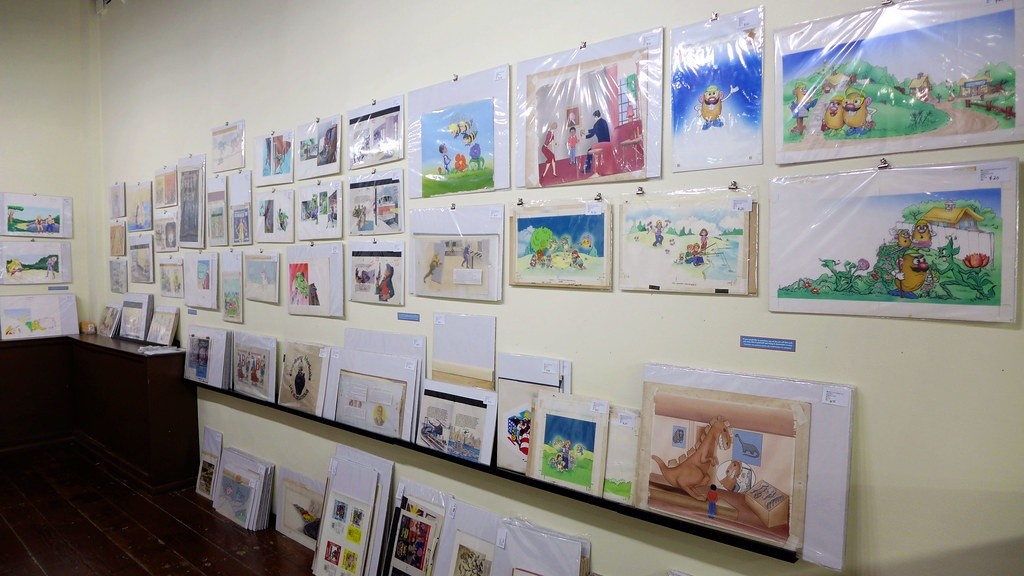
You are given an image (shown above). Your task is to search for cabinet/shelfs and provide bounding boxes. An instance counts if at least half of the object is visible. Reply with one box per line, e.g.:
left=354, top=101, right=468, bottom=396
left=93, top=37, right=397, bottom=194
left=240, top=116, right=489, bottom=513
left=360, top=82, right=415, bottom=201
left=0, top=332, right=200, bottom=494
